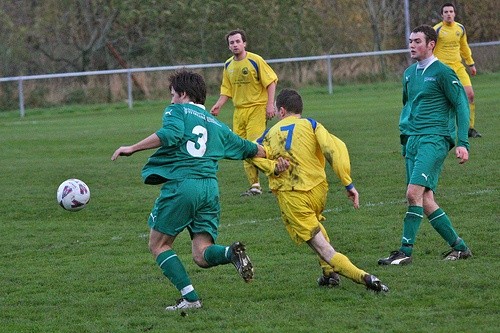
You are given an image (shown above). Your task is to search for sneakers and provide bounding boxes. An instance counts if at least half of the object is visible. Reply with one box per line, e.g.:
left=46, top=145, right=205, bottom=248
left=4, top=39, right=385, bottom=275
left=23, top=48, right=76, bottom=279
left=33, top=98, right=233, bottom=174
left=379, top=251, right=412, bottom=266
left=365, top=274, right=388, bottom=293
left=441, top=248, right=472, bottom=261
left=230, top=241, right=254, bottom=282
left=468, top=129, right=480, bottom=138
left=317, top=270, right=340, bottom=287
left=166, top=299, right=201, bottom=311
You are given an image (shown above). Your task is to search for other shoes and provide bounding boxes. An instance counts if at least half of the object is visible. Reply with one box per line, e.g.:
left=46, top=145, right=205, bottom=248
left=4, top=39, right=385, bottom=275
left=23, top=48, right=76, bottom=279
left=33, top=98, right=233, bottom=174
left=240, top=188, right=262, bottom=198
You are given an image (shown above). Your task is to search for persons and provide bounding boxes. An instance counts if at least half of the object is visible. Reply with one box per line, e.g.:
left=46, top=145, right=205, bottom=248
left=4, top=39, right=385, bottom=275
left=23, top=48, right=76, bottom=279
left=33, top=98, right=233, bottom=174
left=111, top=70, right=266, bottom=312
left=377, top=24, right=474, bottom=266
left=245, top=90, right=389, bottom=294
left=210, top=30, right=278, bottom=197
left=430, top=3, right=482, bottom=138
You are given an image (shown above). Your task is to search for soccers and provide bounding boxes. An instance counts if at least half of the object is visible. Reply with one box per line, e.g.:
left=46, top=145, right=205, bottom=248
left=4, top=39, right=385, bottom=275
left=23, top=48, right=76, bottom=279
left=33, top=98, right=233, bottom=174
left=56, top=178, right=91, bottom=212
